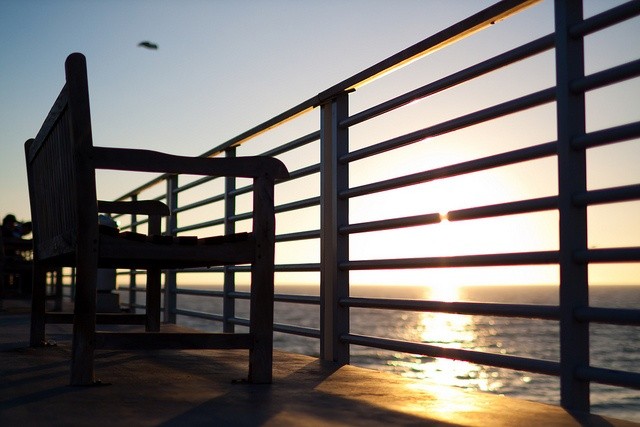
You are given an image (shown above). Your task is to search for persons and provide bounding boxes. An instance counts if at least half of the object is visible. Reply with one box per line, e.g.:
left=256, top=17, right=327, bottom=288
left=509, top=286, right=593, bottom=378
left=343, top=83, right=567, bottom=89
left=0, top=213, right=35, bottom=296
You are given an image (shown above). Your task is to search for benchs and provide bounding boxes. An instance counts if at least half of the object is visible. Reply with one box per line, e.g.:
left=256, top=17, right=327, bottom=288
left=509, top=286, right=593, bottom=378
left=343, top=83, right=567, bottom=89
left=23, top=52, right=289, bottom=391
